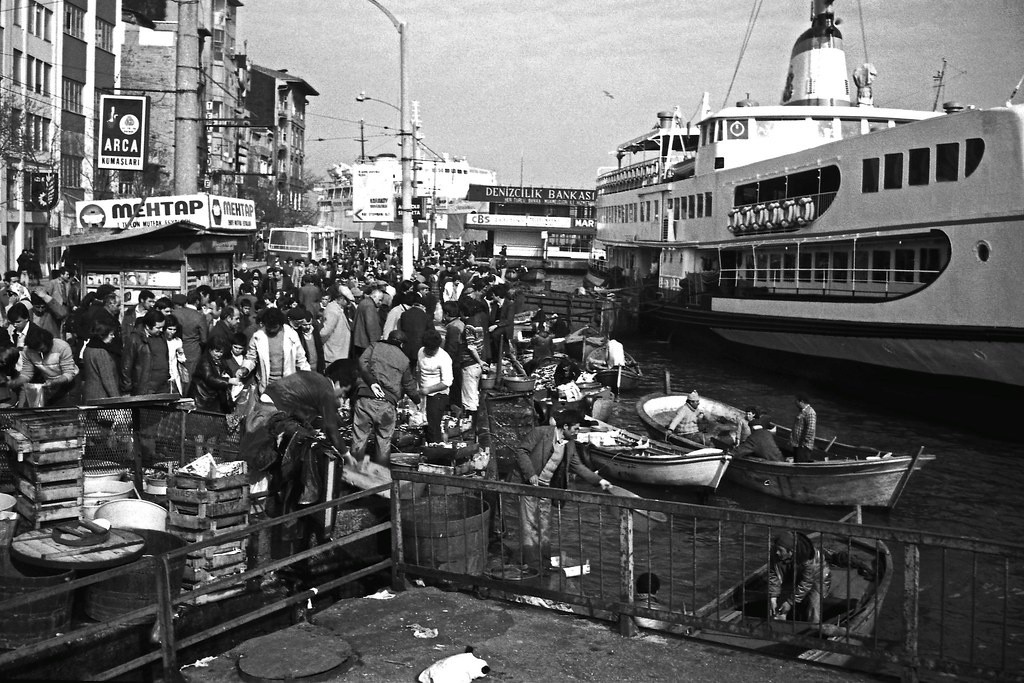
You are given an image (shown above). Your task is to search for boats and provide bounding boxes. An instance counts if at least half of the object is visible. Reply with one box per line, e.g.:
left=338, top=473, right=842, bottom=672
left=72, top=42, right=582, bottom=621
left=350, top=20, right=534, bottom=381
left=580, top=0, right=1023, bottom=444
left=513, top=307, right=734, bottom=495
left=636, top=366, right=937, bottom=515
left=685, top=531, right=894, bottom=667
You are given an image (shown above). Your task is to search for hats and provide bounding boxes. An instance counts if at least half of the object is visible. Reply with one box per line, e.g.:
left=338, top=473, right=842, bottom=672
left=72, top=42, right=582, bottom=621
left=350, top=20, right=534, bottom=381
left=775, top=530, right=794, bottom=550
left=688, top=390, right=699, bottom=401
left=748, top=419, right=762, bottom=426
left=377, top=280, right=389, bottom=286
left=417, top=283, right=430, bottom=289
left=339, top=286, right=355, bottom=302
left=389, top=330, right=408, bottom=342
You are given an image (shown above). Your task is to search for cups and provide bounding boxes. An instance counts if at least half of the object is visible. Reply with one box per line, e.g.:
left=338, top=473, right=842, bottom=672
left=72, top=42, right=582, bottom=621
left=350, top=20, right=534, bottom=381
left=23, top=382, right=49, bottom=408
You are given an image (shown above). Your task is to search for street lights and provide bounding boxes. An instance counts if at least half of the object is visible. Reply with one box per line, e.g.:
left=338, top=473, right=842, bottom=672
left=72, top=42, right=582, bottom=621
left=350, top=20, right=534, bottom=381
left=354, top=0, right=421, bottom=279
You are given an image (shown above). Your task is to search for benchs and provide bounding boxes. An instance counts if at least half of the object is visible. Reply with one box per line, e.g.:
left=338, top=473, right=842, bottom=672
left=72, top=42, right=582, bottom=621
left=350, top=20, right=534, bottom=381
left=746, top=566, right=871, bottom=603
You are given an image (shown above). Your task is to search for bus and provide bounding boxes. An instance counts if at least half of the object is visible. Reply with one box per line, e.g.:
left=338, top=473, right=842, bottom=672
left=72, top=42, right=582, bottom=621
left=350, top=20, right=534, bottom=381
left=267, top=225, right=344, bottom=268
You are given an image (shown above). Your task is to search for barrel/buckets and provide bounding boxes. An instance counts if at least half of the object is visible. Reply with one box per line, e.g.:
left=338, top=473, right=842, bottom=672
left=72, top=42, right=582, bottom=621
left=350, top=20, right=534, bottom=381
left=400, top=492, right=491, bottom=576
left=83, top=524, right=186, bottom=622
left=0, top=510, right=19, bottom=547
left=0, top=545, right=77, bottom=651
left=83, top=479, right=172, bottom=533
left=0, top=492, right=18, bottom=512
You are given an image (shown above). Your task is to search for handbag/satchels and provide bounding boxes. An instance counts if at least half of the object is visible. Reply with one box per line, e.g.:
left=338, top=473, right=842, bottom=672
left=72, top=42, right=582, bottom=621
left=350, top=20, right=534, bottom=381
left=178, top=362, right=189, bottom=383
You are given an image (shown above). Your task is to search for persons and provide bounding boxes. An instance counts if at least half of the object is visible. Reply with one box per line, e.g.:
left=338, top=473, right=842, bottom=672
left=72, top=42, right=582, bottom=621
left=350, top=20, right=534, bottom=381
left=790, top=395, right=817, bottom=462
left=522, top=307, right=581, bottom=385
left=412, top=239, right=508, bottom=284
left=232, top=256, right=342, bottom=318
left=514, top=408, right=613, bottom=570
left=633, top=573, right=670, bottom=611
left=0, top=249, right=126, bottom=462
left=769, top=531, right=833, bottom=624
left=342, top=233, right=402, bottom=283
left=727, top=406, right=785, bottom=462
left=251, top=235, right=269, bottom=262
left=663, top=389, right=710, bottom=444
left=602, top=330, right=625, bottom=369
left=320, top=275, right=515, bottom=378
left=120, top=284, right=311, bottom=460
left=240, top=301, right=482, bottom=548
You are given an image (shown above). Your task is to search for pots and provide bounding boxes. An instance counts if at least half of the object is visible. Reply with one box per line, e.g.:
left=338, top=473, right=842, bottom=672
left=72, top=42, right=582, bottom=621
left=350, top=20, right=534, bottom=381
left=390, top=453, right=428, bottom=471
left=481, top=373, right=496, bottom=389
left=503, top=377, right=535, bottom=391
